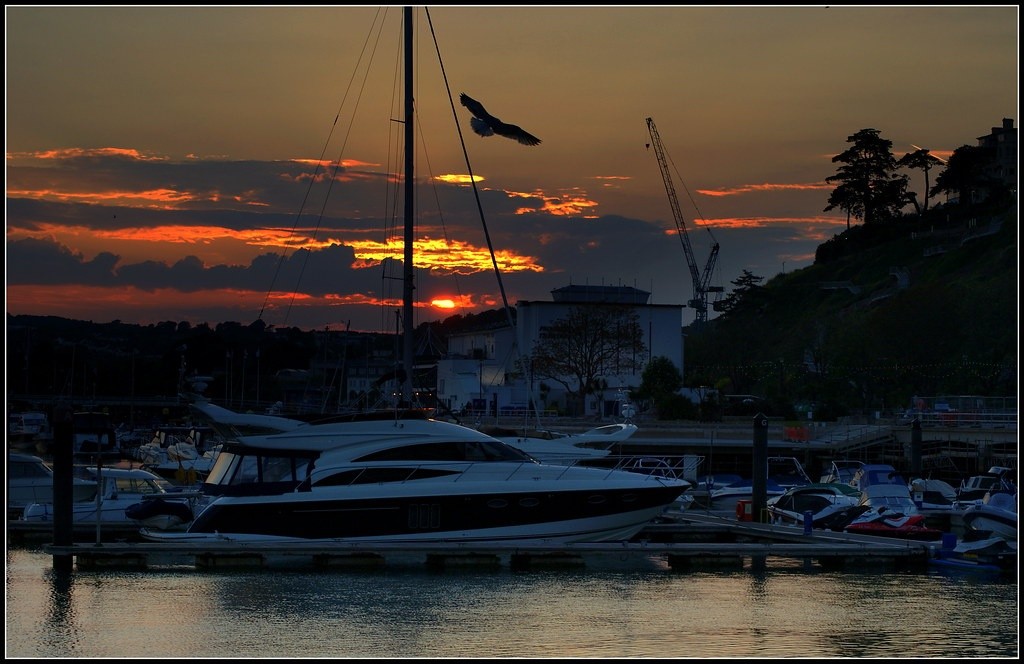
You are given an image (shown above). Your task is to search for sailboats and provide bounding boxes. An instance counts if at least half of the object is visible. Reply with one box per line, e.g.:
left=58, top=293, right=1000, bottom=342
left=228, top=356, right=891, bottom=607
left=4, top=7, right=1018, bottom=578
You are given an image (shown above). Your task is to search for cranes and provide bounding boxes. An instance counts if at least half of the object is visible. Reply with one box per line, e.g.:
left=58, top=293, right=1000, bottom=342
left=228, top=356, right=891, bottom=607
left=646, top=116, right=724, bottom=331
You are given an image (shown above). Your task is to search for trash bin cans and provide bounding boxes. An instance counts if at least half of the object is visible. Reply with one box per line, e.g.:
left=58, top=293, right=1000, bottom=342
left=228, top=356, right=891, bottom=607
left=944, top=412, right=958, bottom=427
left=736, top=501, right=753, bottom=522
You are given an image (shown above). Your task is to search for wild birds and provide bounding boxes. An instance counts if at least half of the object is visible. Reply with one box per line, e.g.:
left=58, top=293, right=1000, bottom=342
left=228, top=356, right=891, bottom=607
left=459, top=91, right=543, bottom=147
